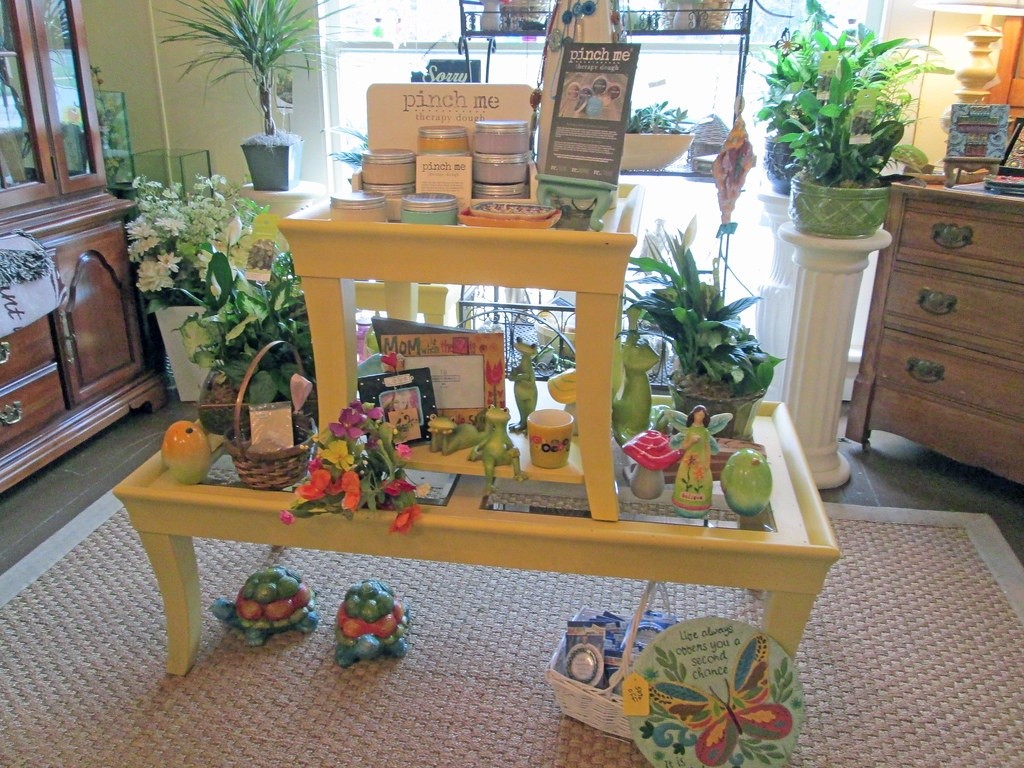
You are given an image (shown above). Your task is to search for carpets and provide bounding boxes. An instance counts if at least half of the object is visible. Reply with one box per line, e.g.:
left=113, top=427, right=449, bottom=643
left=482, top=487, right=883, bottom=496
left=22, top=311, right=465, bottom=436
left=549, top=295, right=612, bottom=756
left=0, top=488, right=1024, bottom=768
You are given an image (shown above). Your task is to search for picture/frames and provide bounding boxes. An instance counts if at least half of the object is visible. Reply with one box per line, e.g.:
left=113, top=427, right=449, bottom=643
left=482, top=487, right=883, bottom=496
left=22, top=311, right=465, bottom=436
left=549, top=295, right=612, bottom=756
left=382, top=334, right=506, bottom=425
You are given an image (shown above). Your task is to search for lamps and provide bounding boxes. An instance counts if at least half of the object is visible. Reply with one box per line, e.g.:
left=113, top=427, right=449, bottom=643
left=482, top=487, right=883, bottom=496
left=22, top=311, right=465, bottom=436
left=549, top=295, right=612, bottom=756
left=913, top=0, right=1024, bottom=184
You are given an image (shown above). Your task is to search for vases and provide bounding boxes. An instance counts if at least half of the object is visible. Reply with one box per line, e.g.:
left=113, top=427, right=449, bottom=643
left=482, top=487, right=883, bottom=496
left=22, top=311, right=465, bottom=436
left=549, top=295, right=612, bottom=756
left=152, top=305, right=211, bottom=403
left=198, top=370, right=318, bottom=438
left=665, top=376, right=766, bottom=444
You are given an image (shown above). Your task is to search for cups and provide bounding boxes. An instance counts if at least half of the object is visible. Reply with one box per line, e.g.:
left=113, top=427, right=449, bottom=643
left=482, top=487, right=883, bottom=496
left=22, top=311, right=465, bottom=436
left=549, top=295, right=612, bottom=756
left=526, top=409, right=574, bottom=468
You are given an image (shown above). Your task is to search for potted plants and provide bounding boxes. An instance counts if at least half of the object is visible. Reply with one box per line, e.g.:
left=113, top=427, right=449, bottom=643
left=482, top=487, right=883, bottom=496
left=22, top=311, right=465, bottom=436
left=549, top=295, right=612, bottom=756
left=619, top=101, right=696, bottom=171
left=152, top=0, right=374, bottom=192
left=752, top=0, right=955, bottom=241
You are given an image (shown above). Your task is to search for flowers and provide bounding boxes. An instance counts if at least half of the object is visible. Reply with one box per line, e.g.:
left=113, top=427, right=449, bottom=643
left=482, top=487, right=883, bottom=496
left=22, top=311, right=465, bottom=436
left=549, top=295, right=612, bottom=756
left=276, top=402, right=429, bottom=535
left=614, top=216, right=787, bottom=397
left=87, top=59, right=127, bottom=185
left=123, top=174, right=314, bottom=402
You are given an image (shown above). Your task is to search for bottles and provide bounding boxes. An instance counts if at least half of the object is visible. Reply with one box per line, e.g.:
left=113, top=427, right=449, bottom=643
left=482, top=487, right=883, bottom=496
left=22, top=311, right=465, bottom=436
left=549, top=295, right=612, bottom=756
left=480, top=0, right=500, bottom=31
left=672, top=0, right=696, bottom=30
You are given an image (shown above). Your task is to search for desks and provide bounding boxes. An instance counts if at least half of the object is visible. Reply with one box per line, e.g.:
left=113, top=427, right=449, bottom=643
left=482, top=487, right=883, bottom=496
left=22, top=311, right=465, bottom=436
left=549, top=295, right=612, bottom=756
left=275, top=178, right=645, bottom=522
left=110, top=400, right=840, bottom=675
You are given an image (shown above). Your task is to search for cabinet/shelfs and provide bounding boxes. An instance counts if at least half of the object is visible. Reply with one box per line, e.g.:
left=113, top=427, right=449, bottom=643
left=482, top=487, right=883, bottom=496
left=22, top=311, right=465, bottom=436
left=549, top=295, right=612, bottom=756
left=457, top=0, right=754, bottom=393
left=843, top=177, right=1024, bottom=486
left=0, top=0, right=167, bottom=499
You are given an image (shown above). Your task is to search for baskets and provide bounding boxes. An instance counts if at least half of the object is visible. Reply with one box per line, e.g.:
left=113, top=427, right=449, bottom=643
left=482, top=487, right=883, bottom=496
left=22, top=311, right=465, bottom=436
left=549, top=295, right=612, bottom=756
left=224, top=341, right=318, bottom=490
left=543, top=579, right=671, bottom=740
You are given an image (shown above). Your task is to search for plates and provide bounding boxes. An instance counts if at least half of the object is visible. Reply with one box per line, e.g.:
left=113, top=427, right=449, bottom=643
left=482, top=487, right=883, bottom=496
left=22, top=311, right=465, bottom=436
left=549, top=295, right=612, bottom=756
left=457, top=202, right=562, bottom=229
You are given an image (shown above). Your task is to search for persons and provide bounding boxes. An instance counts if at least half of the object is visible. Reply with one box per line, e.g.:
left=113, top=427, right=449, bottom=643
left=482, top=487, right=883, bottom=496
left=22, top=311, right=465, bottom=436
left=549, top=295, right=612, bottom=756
left=672, top=404, right=713, bottom=518
